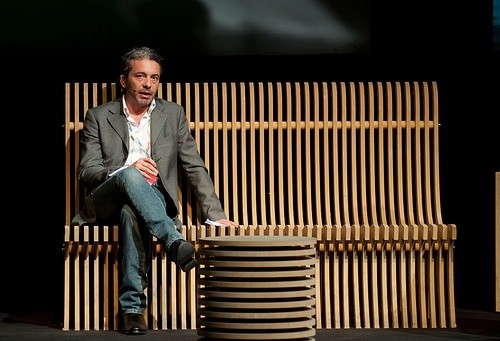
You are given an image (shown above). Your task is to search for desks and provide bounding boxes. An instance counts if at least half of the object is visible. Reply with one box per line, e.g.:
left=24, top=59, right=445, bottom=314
left=196, top=235, right=317, bottom=341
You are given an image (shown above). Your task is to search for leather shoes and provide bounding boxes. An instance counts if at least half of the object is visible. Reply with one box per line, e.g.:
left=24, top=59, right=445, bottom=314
left=121, top=313, right=148, bottom=334
left=171, top=239, right=199, bottom=272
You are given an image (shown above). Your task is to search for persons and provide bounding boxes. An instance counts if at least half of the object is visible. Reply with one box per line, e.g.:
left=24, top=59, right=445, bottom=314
left=79, top=44, right=239, bottom=337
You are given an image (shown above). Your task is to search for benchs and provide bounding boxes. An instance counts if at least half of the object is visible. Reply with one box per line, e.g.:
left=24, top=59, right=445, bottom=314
left=63, top=83, right=458, bottom=333
